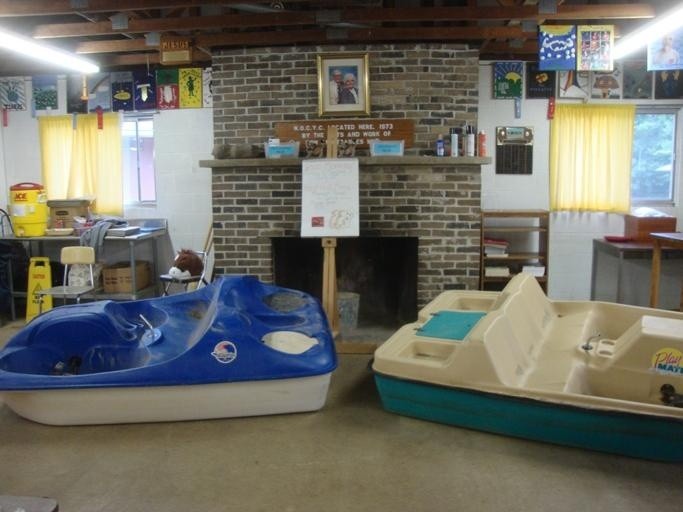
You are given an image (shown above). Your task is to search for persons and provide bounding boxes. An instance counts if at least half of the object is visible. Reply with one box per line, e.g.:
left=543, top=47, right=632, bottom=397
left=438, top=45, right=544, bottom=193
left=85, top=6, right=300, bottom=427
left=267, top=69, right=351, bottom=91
left=330, top=69, right=343, bottom=105
left=671, top=70, right=683, bottom=98
left=653, top=35, right=680, bottom=65
left=186, top=76, right=197, bottom=96
left=339, top=73, right=359, bottom=104
left=656, top=71, right=673, bottom=98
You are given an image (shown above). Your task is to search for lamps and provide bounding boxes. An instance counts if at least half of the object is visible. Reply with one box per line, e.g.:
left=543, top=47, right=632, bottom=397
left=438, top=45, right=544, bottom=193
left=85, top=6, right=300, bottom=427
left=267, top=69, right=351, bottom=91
left=111, top=13, right=129, bottom=30
left=0, top=27, right=100, bottom=75
left=145, top=32, right=162, bottom=46
left=605, top=4, right=683, bottom=62
left=71, top=0, right=88, bottom=9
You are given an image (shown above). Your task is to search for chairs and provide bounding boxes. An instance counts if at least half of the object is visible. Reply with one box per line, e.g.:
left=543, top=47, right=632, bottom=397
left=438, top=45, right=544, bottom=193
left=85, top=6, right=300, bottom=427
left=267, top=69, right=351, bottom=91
left=160, top=249, right=207, bottom=296
left=35, top=246, right=96, bottom=316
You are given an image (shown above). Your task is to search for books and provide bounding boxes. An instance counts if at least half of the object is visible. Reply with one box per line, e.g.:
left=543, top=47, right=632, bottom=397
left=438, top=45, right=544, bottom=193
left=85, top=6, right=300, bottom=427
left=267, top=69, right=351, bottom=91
left=107, top=226, right=140, bottom=236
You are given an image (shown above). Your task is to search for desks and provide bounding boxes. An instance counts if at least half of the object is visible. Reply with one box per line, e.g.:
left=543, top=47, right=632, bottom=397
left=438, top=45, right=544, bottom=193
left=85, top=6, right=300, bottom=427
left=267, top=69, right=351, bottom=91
left=590, top=239, right=683, bottom=311
left=0, top=229, right=165, bottom=320
left=649, top=232, right=683, bottom=312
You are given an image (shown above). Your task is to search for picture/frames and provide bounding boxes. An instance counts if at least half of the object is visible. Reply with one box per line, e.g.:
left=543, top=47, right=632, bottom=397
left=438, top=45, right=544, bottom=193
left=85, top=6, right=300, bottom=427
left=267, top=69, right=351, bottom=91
left=316, top=53, right=371, bottom=117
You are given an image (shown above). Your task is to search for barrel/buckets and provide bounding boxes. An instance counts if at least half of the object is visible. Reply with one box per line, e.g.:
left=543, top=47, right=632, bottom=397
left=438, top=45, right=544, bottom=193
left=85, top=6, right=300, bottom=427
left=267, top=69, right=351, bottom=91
left=10, top=182, right=49, bottom=237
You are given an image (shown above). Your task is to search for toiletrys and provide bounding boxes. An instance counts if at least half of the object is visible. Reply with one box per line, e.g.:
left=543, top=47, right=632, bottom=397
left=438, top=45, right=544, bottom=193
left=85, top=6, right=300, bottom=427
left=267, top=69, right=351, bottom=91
left=435, top=134, right=445, bottom=156
left=450, top=127, right=458, bottom=157
left=478, top=131, right=487, bottom=158
left=464, top=124, right=475, bottom=157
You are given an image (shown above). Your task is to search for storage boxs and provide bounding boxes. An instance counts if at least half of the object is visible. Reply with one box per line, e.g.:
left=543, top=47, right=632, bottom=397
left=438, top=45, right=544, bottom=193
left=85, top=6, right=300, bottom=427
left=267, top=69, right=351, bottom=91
left=101, top=260, right=152, bottom=293
left=50, top=207, right=88, bottom=230
left=367, top=138, right=405, bottom=156
left=263, top=140, right=300, bottom=159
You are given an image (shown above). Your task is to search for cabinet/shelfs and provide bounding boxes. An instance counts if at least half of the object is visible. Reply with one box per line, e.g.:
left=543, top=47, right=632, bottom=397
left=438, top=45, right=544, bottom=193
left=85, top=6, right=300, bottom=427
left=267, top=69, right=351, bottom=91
left=480, top=211, right=549, bottom=297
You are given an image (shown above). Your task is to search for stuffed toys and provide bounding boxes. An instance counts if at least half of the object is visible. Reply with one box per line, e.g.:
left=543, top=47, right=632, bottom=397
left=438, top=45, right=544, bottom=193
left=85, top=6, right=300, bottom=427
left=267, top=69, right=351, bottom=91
left=169, top=248, right=203, bottom=292
